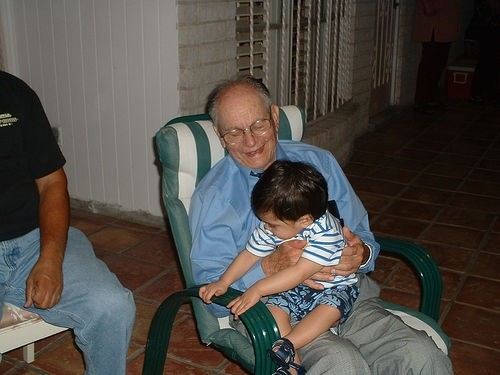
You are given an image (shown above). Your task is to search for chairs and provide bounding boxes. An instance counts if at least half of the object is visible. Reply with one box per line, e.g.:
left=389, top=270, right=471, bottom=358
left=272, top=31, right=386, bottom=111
left=142, top=106, right=451, bottom=375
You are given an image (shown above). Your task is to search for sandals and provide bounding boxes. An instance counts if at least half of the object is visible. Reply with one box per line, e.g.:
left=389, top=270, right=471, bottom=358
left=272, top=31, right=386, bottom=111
left=272, top=362, right=307, bottom=375
left=271, top=338, right=295, bottom=368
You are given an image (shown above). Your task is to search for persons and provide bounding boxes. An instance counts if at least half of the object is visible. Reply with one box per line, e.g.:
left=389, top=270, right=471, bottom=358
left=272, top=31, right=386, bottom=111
left=0, top=70, right=136, bottom=375
left=200, top=160, right=360, bottom=374
left=189, top=74, right=456, bottom=375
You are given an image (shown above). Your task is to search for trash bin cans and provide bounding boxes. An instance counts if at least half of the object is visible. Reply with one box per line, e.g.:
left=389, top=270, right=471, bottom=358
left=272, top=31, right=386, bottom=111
left=446, top=66, right=475, bottom=103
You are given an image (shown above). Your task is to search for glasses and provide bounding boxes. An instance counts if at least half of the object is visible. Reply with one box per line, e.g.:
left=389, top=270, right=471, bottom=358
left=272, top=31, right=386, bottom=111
left=218, top=105, right=272, bottom=145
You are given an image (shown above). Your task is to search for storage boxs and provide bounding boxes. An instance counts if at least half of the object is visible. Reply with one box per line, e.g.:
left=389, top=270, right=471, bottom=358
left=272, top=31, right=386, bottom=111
left=447, top=66, right=475, bottom=99
